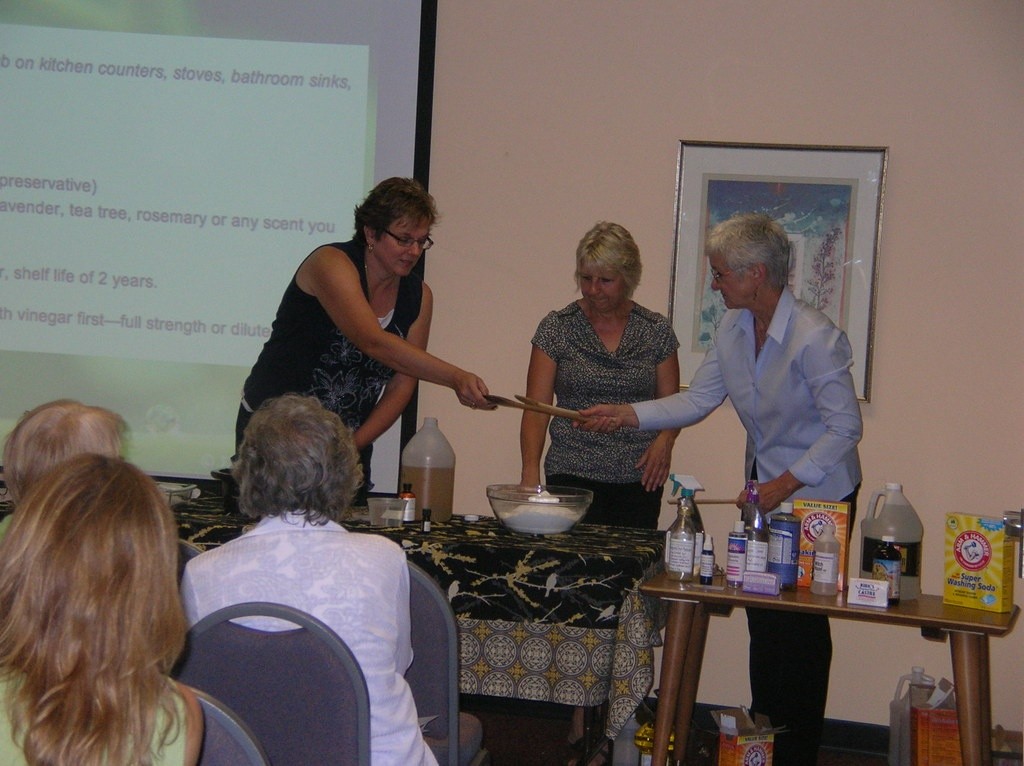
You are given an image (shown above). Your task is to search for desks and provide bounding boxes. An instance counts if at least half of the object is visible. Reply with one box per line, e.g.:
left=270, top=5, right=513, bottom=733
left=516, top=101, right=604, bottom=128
left=0, top=466, right=667, bottom=766
left=641, top=567, right=1019, bottom=766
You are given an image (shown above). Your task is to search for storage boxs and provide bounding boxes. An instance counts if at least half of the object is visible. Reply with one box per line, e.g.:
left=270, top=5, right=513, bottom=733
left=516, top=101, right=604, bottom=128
left=794, top=498, right=851, bottom=590
left=945, top=512, right=1014, bottom=613
left=709, top=704, right=789, bottom=766
left=908, top=677, right=962, bottom=766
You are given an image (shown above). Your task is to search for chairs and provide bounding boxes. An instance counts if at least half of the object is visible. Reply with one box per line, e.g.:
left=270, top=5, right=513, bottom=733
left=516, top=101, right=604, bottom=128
left=170, top=603, right=372, bottom=766
left=404, top=557, right=483, bottom=766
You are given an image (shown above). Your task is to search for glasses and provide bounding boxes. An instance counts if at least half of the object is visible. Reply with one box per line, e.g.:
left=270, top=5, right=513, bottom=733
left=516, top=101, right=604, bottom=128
left=382, top=229, right=433, bottom=250
left=710, top=269, right=745, bottom=281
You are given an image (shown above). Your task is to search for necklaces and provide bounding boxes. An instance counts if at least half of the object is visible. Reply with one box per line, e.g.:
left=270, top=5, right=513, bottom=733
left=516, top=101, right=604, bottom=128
left=364, top=260, right=374, bottom=298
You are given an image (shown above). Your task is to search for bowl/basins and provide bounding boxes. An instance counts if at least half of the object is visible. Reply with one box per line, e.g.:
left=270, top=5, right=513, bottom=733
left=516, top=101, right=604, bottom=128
left=366, top=497, right=409, bottom=527
left=485, top=484, right=594, bottom=534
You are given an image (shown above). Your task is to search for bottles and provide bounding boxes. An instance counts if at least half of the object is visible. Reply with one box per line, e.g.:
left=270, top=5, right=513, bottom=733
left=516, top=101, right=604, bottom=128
left=809, top=524, right=841, bottom=596
left=668, top=490, right=715, bottom=585
left=769, top=502, right=802, bottom=591
left=398, top=482, right=416, bottom=526
left=612, top=698, right=679, bottom=766
left=859, top=481, right=925, bottom=606
left=421, top=508, right=432, bottom=533
left=886, top=664, right=936, bottom=765
left=399, top=416, right=456, bottom=522
left=725, top=520, right=748, bottom=589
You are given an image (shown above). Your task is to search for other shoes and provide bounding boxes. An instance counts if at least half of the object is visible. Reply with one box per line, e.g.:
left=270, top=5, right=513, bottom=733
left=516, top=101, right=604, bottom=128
left=562, top=729, right=602, bottom=766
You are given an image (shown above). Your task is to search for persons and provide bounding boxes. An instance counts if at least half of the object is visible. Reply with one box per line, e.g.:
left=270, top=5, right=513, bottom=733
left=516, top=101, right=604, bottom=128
left=0, top=453, right=204, bottom=766
left=236, top=176, right=497, bottom=493
left=567, top=217, right=863, bottom=766
left=0, top=399, right=132, bottom=540
left=516, top=221, right=680, bottom=766
left=173, top=393, right=439, bottom=766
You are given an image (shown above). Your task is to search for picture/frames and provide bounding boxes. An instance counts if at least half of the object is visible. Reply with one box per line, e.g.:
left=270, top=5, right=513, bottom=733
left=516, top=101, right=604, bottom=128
left=666, top=138, right=891, bottom=404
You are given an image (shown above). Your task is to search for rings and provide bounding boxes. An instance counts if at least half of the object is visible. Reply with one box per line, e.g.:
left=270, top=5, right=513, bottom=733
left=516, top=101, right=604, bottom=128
left=470, top=405, right=477, bottom=408
